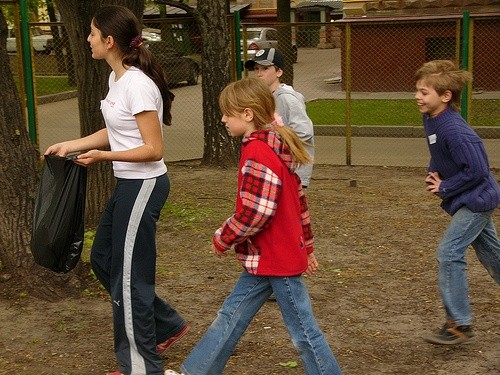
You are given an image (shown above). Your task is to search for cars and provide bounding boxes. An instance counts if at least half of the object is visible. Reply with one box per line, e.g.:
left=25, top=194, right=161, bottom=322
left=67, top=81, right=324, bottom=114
left=6, top=25, right=56, bottom=55
left=141, top=41, right=200, bottom=89
left=140, top=31, right=163, bottom=43
left=239, top=28, right=298, bottom=71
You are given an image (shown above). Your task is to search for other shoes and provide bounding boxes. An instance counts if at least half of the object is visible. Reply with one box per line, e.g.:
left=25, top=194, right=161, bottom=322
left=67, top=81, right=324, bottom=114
left=423, top=322, right=475, bottom=346
left=156, top=323, right=191, bottom=356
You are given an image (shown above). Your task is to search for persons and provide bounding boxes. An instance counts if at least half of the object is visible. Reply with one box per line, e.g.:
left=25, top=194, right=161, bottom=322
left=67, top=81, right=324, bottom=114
left=164, top=77, right=342, bottom=375
left=41, top=4, right=190, bottom=375
left=413, top=59, right=499, bottom=345
left=244, top=48, right=315, bottom=194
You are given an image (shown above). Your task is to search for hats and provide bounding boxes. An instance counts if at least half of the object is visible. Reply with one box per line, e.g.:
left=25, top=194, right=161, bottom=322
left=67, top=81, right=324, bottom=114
left=245, top=48, right=284, bottom=70
